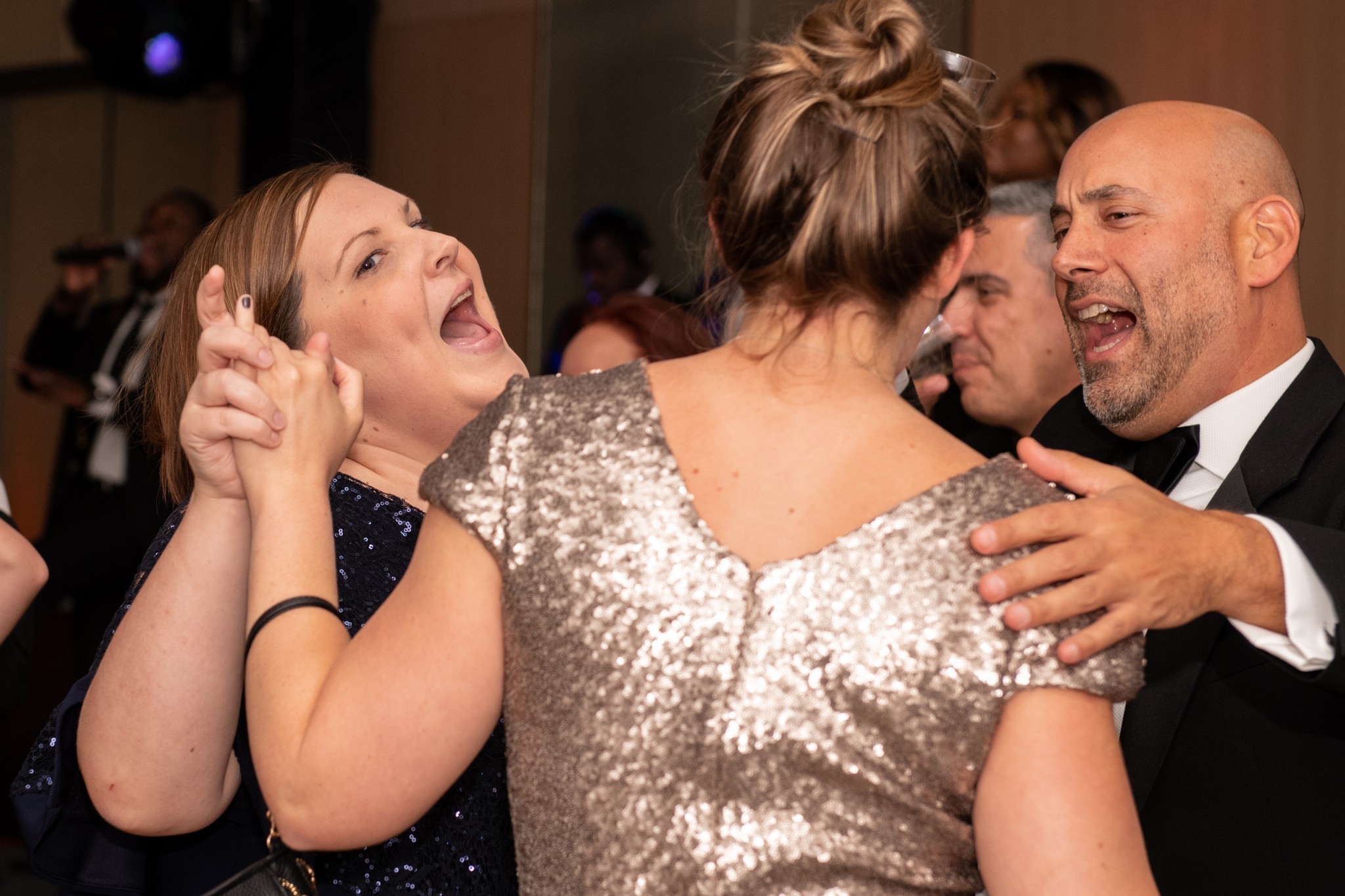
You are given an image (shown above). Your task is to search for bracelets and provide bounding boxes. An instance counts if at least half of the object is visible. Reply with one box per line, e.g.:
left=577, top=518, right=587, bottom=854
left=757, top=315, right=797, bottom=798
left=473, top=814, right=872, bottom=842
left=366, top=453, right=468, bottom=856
left=242, top=596, right=343, bottom=665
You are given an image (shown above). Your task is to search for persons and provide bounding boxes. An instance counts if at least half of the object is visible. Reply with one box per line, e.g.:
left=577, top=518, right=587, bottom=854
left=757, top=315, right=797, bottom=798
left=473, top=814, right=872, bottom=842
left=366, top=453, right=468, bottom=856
left=521, top=61, right=1144, bottom=497
left=1, top=191, right=219, bottom=801
left=232, top=1, right=1161, bottom=895
left=0, top=163, right=532, bottom=896
left=969, top=99, right=1345, bottom=896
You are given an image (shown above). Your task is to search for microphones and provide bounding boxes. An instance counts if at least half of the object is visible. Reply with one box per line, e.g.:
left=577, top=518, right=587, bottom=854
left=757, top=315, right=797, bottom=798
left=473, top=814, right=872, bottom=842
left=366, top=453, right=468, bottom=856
left=53, top=241, right=141, bottom=264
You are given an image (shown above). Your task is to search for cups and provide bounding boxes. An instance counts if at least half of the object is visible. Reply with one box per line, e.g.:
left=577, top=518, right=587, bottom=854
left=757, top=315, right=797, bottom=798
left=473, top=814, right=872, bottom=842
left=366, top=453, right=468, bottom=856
left=905, top=315, right=966, bottom=379
